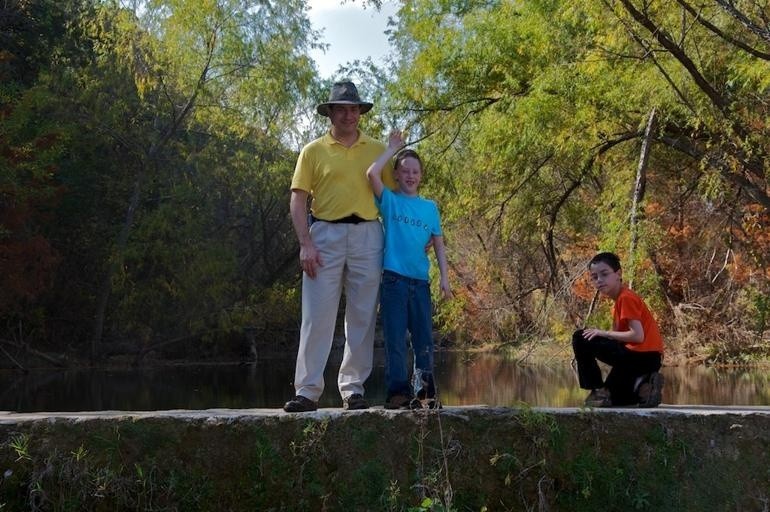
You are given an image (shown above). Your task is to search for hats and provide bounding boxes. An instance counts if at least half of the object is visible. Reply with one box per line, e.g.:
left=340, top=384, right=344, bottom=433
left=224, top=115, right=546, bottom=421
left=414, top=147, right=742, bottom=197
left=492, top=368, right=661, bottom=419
left=315, top=81, right=374, bottom=115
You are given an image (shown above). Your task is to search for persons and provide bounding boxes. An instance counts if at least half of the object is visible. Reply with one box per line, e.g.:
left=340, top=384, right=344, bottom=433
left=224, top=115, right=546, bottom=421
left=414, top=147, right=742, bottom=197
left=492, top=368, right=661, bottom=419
left=366, top=130, right=456, bottom=412
left=570, top=253, right=666, bottom=409
left=282, top=79, right=396, bottom=415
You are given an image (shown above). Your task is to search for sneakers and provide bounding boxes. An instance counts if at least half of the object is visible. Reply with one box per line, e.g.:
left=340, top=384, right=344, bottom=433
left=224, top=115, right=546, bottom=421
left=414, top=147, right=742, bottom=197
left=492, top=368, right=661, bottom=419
left=583, top=388, right=614, bottom=408
left=383, top=392, right=441, bottom=409
left=637, top=371, right=665, bottom=408
left=284, top=395, right=319, bottom=412
left=343, top=394, right=372, bottom=409
